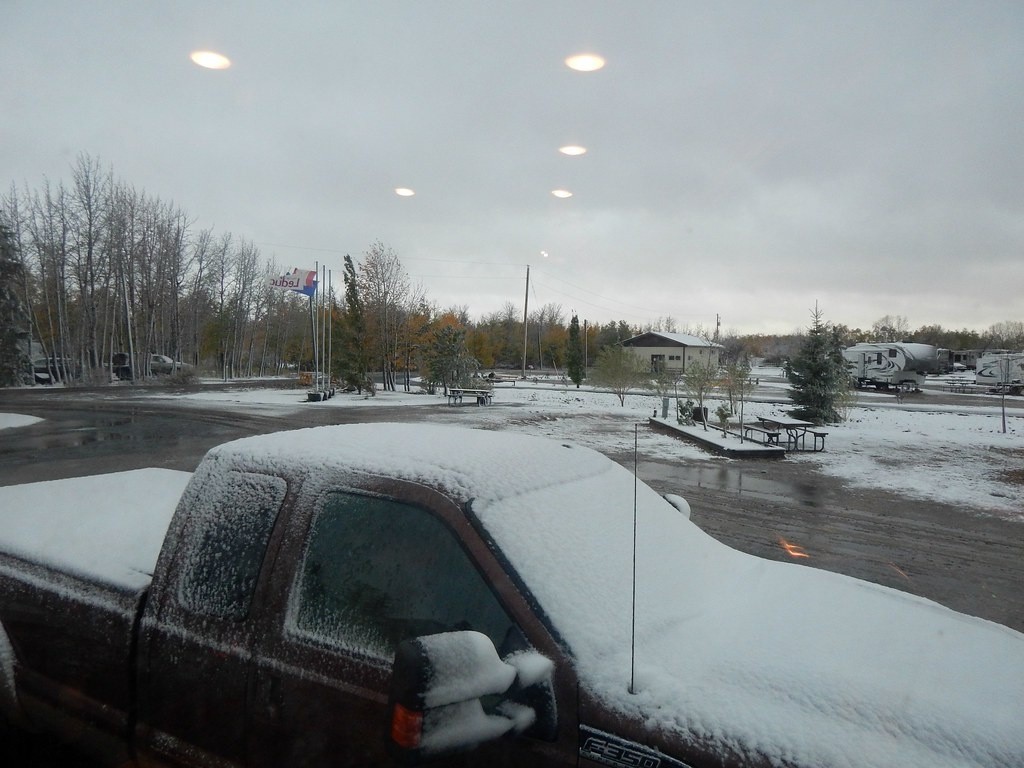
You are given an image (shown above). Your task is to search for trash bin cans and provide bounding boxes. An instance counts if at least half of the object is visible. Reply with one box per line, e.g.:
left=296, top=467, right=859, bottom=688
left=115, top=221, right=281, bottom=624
left=692, top=407, right=708, bottom=421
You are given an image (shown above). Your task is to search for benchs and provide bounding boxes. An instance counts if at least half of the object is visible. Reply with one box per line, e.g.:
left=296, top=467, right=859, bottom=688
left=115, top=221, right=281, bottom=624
left=788, top=427, right=828, bottom=453
left=446, top=394, right=493, bottom=407
left=942, top=386, right=976, bottom=394
left=743, top=424, right=781, bottom=443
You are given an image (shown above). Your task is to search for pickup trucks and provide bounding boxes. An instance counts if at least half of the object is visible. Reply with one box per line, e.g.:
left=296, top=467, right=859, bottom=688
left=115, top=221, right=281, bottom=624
left=0, top=420, right=1024, bottom=768
left=104, top=354, right=195, bottom=382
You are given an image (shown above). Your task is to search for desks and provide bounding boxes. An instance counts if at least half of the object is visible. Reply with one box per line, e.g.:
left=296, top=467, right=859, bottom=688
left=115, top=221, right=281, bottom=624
left=945, top=381, right=974, bottom=393
left=997, top=383, right=1024, bottom=395
left=756, top=416, right=814, bottom=453
left=448, top=389, right=492, bottom=407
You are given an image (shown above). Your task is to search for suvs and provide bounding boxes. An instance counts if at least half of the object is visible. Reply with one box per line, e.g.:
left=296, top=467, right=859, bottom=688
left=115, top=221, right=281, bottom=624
left=23, top=356, right=84, bottom=384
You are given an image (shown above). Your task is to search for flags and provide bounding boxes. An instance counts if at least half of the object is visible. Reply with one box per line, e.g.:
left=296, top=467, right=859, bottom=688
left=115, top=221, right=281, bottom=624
left=265, top=268, right=320, bottom=298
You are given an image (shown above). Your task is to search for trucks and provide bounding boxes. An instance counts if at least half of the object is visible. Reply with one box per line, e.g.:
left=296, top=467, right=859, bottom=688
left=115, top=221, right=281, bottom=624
left=818, top=339, right=1024, bottom=397
